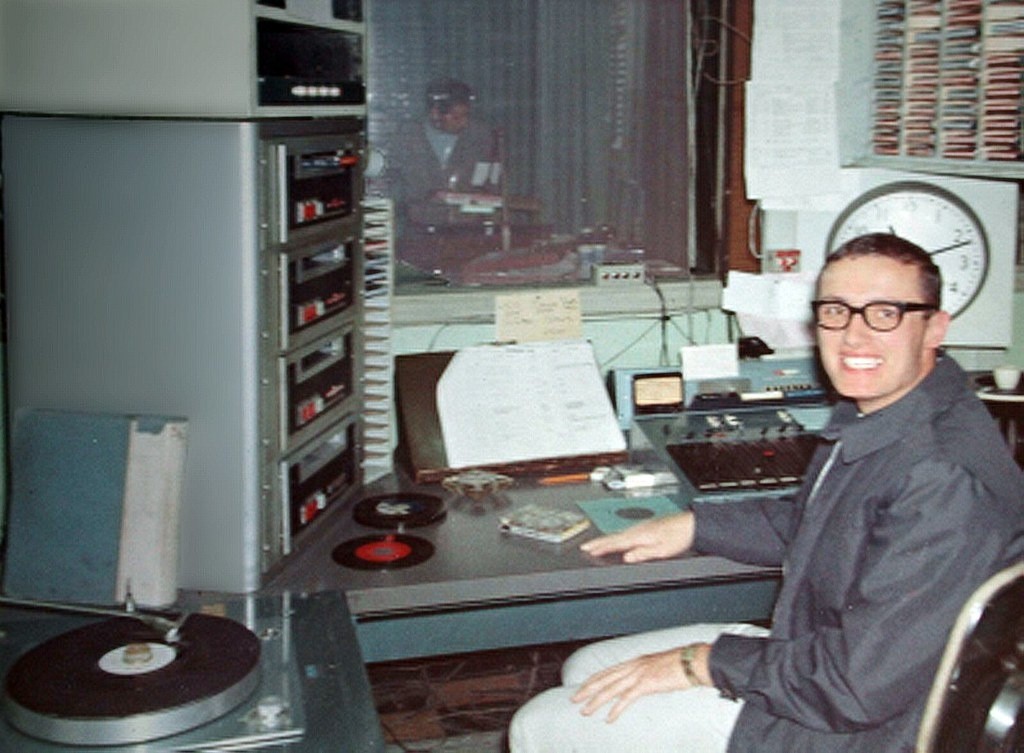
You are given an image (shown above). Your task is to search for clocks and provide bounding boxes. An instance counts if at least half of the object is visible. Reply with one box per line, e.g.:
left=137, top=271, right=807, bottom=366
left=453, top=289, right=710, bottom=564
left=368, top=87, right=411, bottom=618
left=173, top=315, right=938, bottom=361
left=826, top=180, right=991, bottom=321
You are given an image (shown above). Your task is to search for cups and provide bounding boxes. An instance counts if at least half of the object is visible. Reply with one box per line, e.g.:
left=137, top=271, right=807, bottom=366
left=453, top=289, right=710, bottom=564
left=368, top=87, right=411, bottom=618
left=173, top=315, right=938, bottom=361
left=993, top=365, right=1021, bottom=390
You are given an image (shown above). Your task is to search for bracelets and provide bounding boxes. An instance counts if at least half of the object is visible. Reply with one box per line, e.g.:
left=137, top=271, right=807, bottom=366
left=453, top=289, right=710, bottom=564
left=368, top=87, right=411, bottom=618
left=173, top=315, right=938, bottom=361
left=682, top=643, right=705, bottom=686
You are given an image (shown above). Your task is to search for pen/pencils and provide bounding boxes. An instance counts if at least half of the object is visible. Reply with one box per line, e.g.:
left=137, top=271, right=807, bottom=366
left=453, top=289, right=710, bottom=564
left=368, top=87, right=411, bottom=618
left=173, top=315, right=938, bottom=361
left=538, top=475, right=590, bottom=483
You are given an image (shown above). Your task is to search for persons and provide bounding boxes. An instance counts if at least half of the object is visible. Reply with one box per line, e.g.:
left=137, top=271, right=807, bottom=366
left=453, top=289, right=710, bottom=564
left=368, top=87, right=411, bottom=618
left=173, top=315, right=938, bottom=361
left=383, top=79, right=503, bottom=263
left=508, top=232, right=1024, bottom=753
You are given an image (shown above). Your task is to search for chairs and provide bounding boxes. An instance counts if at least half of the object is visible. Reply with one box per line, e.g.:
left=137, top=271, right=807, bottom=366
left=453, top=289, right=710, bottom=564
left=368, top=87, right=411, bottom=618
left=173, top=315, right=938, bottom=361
left=915, top=561, right=1024, bottom=753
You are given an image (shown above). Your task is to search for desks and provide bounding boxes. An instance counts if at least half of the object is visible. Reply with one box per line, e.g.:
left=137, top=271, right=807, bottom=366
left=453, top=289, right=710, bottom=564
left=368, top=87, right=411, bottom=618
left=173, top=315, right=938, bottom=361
left=272, top=473, right=786, bottom=665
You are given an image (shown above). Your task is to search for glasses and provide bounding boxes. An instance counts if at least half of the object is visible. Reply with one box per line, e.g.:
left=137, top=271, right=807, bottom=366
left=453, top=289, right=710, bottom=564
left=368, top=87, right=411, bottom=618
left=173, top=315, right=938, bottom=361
left=810, top=299, right=939, bottom=332
